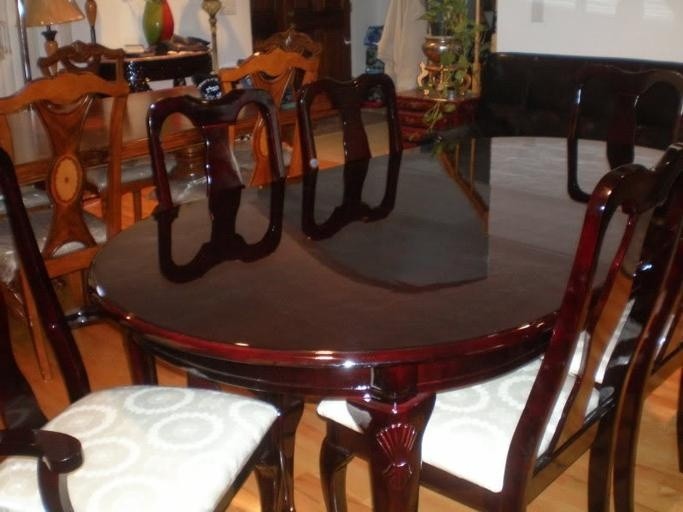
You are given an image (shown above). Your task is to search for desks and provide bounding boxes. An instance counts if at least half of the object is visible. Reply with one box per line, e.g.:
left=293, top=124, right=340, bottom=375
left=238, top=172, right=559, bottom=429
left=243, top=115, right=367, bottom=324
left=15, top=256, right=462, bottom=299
left=0, top=84, right=337, bottom=256
left=98, top=44, right=212, bottom=89
left=88, top=134, right=666, bottom=511
left=397, top=85, right=482, bottom=152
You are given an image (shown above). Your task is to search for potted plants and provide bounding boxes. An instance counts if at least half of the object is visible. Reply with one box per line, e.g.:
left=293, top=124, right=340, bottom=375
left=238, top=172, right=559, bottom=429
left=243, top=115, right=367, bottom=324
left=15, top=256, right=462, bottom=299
left=415, top=3, right=486, bottom=133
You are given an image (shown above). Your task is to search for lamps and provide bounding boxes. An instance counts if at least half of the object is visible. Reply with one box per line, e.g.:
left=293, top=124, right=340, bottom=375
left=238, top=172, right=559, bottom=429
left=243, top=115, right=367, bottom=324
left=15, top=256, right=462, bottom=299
left=24, top=0, right=86, bottom=71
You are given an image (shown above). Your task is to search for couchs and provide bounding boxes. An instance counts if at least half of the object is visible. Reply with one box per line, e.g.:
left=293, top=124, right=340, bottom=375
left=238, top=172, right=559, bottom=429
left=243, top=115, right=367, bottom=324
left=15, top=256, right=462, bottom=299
left=457, top=48, right=683, bottom=153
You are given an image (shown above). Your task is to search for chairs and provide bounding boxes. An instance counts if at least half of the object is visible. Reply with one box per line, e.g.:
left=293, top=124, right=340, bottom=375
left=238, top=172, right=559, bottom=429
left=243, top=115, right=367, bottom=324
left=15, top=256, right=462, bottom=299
left=317, top=148, right=683, bottom=512
left=253, top=31, right=322, bottom=99
left=38, top=41, right=143, bottom=225
left=1, top=71, right=131, bottom=376
left=297, top=71, right=405, bottom=172
left=614, top=200, right=683, bottom=512
left=0, top=147, right=297, bottom=512
left=218, top=50, right=321, bottom=188
left=147, top=87, right=286, bottom=212
